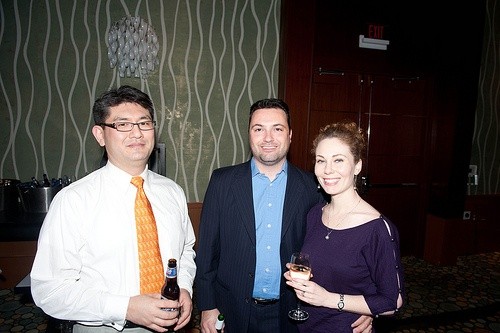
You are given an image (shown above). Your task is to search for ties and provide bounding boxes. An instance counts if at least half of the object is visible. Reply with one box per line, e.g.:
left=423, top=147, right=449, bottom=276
left=130, top=176, right=166, bottom=295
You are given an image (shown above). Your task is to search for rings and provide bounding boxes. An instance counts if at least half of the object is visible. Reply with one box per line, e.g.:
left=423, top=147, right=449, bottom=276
left=302, top=291, right=305, bottom=296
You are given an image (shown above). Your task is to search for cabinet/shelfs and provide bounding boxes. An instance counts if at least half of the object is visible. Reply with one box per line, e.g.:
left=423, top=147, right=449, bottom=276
left=278, top=0, right=479, bottom=256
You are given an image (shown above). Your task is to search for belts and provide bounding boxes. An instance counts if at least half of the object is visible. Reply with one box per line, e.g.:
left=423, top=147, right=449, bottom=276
left=248, top=297, right=279, bottom=305
left=124, top=321, right=140, bottom=328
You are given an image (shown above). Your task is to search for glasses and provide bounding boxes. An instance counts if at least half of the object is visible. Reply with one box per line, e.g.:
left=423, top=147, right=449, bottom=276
left=103, top=121, right=157, bottom=132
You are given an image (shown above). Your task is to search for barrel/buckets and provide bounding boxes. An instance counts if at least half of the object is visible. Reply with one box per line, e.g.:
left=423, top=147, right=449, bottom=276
left=0, top=178, right=21, bottom=214
left=15, top=184, right=63, bottom=213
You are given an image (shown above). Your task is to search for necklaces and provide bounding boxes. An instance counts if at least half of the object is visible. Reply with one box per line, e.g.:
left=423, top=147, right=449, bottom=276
left=326, top=198, right=362, bottom=239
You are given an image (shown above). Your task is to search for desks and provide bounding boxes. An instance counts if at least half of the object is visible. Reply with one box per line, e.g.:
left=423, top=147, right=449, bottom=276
left=0, top=213, right=46, bottom=289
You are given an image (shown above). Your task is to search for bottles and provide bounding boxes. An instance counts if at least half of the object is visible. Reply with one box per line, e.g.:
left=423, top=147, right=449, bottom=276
left=214, top=314, right=225, bottom=333
left=160, top=258, right=180, bottom=333
left=31, top=176, right=78, bottom=187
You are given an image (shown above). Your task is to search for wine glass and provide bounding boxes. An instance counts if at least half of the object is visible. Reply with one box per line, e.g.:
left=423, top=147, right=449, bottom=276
left=288, top=252, right=309, bottom=319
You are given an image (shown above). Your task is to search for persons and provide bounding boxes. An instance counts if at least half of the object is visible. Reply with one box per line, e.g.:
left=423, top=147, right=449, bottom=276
left=284, top=123, right=403, bottom=333
left=193, top=99, right=373, bottom=333
left=30, top=87, right=197, bottom=333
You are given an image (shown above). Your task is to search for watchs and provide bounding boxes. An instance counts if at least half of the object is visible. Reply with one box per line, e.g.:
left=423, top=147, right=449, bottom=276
left=338, top=294, right=344, bottom=310
